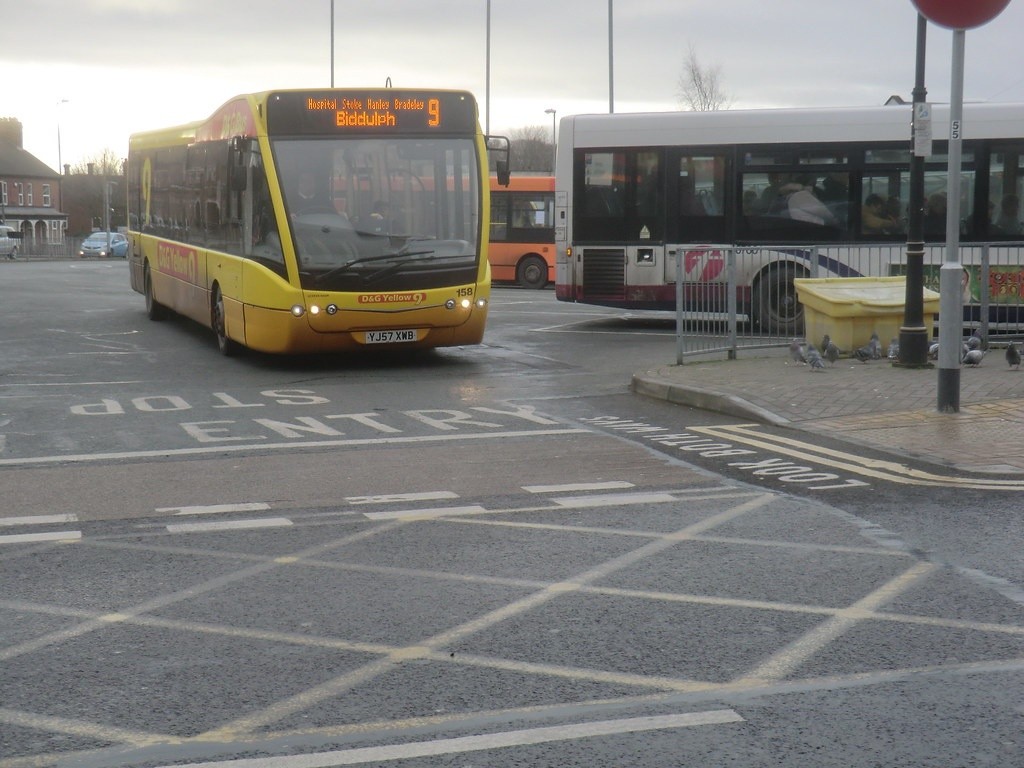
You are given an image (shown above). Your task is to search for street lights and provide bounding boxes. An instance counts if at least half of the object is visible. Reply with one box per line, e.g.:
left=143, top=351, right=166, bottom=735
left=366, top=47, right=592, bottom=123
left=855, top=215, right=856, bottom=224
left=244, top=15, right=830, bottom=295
left=544, top=109, right=556, bottom=178
left=107, top=181, right=118, bottom=258
left=58, top=99, right=69, bottom=212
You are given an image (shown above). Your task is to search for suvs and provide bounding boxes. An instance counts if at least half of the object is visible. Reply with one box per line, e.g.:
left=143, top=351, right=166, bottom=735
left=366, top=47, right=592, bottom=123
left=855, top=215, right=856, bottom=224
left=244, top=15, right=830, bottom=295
left=0, top=226, right=22, bottom=259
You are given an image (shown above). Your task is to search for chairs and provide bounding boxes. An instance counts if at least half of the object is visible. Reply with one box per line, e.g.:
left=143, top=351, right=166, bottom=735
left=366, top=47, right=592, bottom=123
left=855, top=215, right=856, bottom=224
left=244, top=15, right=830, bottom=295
left=586, top=181, right=717, bottom=216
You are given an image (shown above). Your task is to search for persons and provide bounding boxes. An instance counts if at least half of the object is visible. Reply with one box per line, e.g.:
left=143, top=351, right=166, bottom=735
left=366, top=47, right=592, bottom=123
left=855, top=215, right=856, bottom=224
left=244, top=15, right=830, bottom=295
left=861, top=191, right=907, bottom=234
left=753, top=157, right=848, bottom=227
left=289, top=168, right=349, bottom=220
left=924, top=189, right=1023, bottom=236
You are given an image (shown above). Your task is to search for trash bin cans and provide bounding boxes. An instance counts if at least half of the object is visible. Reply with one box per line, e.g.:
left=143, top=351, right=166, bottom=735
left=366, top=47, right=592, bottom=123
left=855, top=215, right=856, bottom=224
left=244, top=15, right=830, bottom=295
left=793, top=276, right=941, bottom=356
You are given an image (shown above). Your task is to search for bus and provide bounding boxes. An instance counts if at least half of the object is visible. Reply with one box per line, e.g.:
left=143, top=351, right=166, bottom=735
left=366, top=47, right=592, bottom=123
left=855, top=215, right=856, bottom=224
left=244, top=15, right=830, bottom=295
left=332, top=171, right=643, bottom=289
left=555, top=104, right=1024, bottom=336
left=127, top=88, right=511, bottom=354
left=91, top=216, right=103, bottom=233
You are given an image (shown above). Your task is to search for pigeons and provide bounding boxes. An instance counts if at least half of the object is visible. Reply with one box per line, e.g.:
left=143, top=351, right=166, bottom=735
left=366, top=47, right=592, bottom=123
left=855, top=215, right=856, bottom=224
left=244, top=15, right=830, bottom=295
left=790, top=331, right=1021, bottom=372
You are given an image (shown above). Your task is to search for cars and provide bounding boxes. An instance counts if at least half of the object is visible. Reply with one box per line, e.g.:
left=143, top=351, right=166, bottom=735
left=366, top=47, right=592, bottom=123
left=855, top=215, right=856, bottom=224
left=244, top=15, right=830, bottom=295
left=79, top=232, right=129, bottom=258
